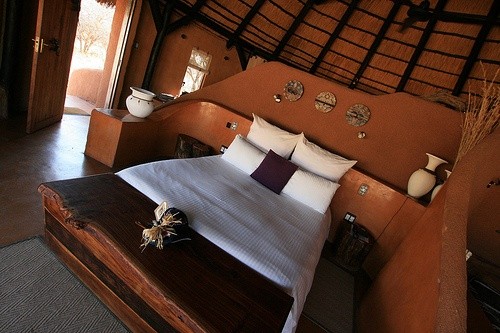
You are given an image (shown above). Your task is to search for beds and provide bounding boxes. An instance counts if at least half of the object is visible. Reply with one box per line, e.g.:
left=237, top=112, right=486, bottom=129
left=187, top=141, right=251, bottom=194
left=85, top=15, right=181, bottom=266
left=115, top=112, right=360, bottom=333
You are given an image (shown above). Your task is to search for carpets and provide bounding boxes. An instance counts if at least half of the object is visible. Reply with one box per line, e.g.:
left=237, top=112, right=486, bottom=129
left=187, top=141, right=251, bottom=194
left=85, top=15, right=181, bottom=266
left=302, top=254, right=355, bottom=333
left=0, top=233, right=130, bottom=333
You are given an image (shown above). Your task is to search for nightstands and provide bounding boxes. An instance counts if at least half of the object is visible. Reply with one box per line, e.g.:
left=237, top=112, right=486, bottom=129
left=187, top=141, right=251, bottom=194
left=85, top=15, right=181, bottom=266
left=84, top=108, right=145, bottom=168
left=321, top=219, right=376, bottom=274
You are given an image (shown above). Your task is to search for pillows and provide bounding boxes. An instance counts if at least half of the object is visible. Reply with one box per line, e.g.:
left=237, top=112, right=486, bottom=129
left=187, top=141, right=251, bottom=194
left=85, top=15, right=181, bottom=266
left=289, top=132, right=358, bottom=183
left=246, top=112, right=301, bottom=160
left=250, top=149, right=299, bottom=195
left=281, top=167, right=341, bottom=215
left=221, top=134, right=267, bottom=175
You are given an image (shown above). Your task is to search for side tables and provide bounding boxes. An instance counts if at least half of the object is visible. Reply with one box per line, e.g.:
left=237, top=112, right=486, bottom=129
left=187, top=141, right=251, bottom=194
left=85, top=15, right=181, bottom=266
left=38, top=172, right=296, bottom=333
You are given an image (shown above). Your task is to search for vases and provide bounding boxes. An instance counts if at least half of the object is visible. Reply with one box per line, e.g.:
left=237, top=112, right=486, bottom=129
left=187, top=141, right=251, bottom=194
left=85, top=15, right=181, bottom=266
left=430, top=169, right=452, bottom=202
left=407, top=152, right=450, bottom=198
left=126, top=86, right=157, bottom=119
